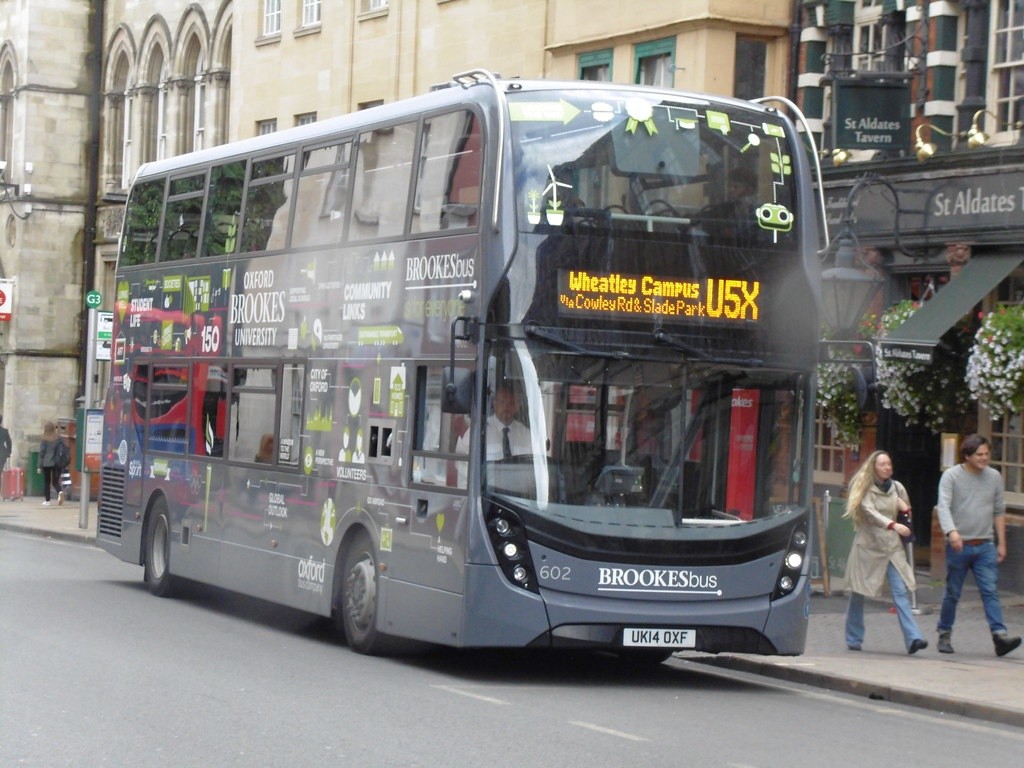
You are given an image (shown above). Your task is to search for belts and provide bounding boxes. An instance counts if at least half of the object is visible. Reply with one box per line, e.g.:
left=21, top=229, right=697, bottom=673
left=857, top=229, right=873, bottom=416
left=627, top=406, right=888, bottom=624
left=963, top=539, right=988, bottom=545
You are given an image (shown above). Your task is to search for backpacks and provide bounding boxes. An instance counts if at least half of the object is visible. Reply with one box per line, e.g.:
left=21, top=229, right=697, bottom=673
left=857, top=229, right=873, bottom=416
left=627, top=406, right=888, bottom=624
left=55, top=438, right=71, bottom=469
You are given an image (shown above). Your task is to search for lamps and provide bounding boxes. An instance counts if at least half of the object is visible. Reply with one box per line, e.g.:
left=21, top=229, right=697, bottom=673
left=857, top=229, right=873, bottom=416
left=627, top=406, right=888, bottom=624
left=832, top=148, right=853, bottom=165
left=914, top=123, right=967, bottom=160
left=967, top=108, right=1023, bottom=149
left=814, top=172, right=925, bottom=346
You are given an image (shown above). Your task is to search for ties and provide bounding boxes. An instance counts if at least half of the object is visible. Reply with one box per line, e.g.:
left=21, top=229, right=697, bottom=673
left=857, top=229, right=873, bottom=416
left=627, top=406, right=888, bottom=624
left=502, top=427, right=512, bottom=457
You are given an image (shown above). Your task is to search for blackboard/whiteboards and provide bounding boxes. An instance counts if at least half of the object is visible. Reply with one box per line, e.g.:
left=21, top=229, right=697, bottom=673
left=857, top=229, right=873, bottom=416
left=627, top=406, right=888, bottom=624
left=767, top=498, right=831, bottom=584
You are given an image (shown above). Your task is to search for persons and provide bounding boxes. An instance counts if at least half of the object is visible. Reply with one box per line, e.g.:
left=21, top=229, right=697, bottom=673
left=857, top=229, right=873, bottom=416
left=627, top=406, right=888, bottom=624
left=844, top=449, right=928, bottom=654
left=454, top=381, right=533, bottom=490
left=0, top=415, right=12, bottom=475
left=241, top=434, right=292, bottom=521
left=936, top=434, right=1022, bottom=656
left=36, top=422, right=64, bottom=506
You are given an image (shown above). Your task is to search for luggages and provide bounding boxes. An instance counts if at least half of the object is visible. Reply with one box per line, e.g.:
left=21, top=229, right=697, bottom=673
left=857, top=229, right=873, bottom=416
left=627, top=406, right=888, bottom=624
left=1, top=454, right=24, bottom=502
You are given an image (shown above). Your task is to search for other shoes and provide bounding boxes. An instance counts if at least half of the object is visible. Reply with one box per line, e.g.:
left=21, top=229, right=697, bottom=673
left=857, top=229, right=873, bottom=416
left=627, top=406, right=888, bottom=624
left=908, top=639, right=928, bottom=654
left=58, top=491, right=64, bottom=505
left=992, top=634, right=1022, bottom=657
left=41, top=501, right=50, bottom=506
left=936, top=633, right=954, bottom=653
left=848, top=645, right=862, bottom=651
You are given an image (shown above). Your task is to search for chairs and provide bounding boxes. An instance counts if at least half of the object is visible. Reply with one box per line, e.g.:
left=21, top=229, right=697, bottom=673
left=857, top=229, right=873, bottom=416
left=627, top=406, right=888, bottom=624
left=261, top=434, right=273, bottom=460
left=150, top=199, right=761, bottom=266
left=604, top=450, right=651, bottom=491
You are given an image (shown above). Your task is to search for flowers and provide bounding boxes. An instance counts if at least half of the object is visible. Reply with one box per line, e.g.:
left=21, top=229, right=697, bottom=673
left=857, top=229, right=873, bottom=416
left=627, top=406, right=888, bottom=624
left=799, top=300, right=1024, bottom=448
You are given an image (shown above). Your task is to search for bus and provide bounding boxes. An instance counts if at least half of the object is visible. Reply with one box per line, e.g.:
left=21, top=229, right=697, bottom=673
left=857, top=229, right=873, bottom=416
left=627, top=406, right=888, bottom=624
left=95, top=68, right=890, bottom=664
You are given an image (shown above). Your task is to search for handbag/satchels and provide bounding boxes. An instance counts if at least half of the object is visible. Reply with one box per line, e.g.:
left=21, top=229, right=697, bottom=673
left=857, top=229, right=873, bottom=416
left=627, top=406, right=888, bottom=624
left=894, top=480, right=916, bottom=544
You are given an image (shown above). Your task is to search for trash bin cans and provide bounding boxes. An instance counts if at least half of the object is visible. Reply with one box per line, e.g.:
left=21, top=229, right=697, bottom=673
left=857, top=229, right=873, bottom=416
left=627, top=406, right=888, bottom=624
left=27, top=449, right=46, bottom=497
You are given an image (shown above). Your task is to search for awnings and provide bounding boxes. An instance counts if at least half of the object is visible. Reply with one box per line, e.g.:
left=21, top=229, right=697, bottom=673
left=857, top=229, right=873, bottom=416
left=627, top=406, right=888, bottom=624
left=880, top=250, right=1024, bottom=365
